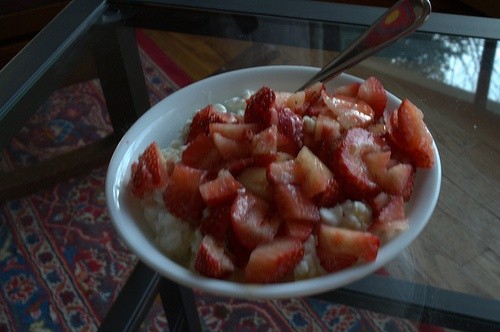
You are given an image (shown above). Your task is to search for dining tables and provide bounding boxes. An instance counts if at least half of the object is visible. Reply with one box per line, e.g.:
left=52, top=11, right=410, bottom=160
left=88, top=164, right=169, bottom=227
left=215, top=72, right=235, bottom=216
left=0, top=1, right=500, bottom=332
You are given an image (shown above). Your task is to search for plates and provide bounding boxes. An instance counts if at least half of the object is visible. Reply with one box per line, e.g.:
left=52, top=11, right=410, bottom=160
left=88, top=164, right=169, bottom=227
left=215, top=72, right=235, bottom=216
left=103, top=64, right=442, bottom=298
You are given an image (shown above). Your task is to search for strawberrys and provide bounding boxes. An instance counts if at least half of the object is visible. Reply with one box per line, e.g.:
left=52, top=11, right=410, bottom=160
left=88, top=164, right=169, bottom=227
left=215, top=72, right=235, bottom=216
left=129, top=77, right=435, bottom=284
